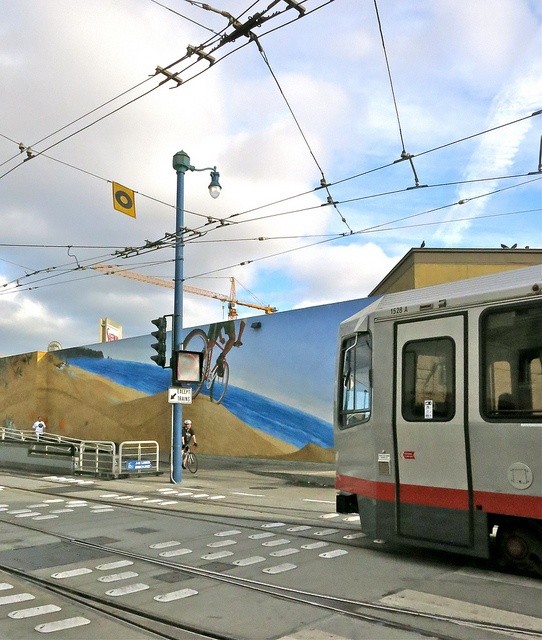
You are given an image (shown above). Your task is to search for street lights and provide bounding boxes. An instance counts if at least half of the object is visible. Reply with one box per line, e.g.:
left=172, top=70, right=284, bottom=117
left=168, top=148, right=224, bottom=483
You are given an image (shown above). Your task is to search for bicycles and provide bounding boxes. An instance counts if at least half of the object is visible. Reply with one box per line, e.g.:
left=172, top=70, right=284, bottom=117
left=168, top=442, right=198, bottom=473
left=180, top=329, right=242, bottom=405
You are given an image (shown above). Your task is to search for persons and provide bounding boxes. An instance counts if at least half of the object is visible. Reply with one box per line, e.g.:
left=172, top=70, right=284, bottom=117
left=181, top=419, right=197, bottom=470
left=32, top=416, right=47, bottom=442
left=201, top=319, right=247, bottom=377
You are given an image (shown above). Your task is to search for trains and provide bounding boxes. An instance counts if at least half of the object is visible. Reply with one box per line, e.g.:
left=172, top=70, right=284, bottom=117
left=326, top=262, right=542, bottom=579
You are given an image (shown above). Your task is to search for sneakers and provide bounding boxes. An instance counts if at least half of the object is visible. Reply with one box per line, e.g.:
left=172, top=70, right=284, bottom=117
left=216, top=353, right=225, bottom=377
left=182, top=462, right=187, bottom=470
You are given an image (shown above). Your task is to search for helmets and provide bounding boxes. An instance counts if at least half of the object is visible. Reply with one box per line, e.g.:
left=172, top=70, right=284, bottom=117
left=183, top=419, right=192, bottom=425
